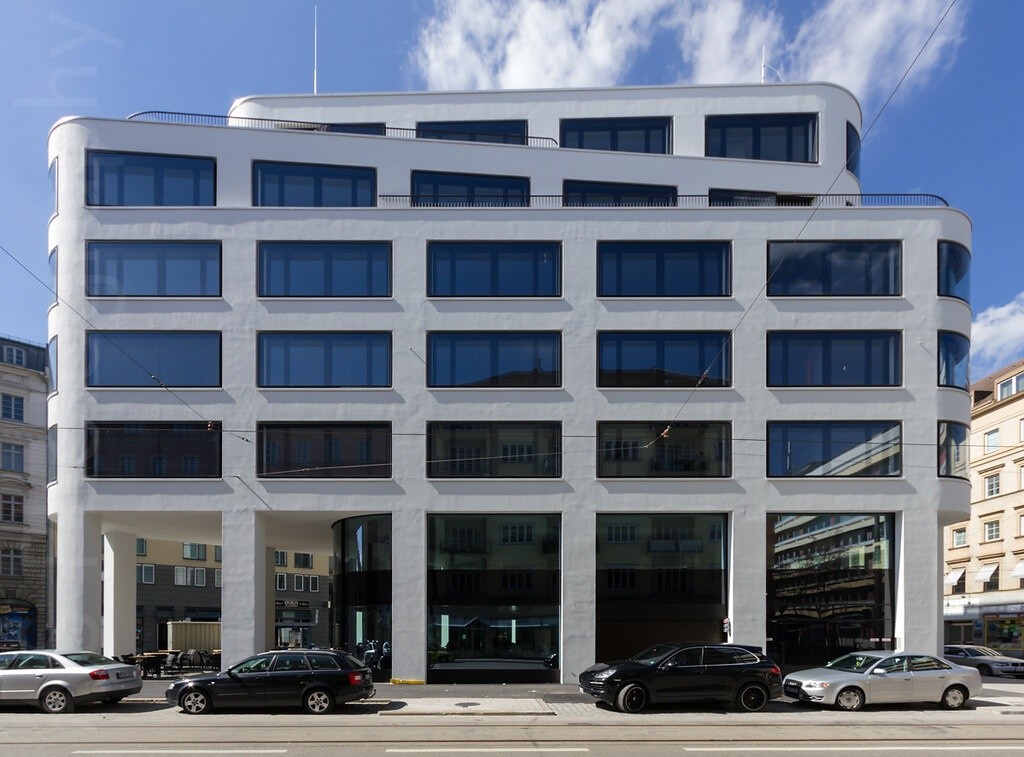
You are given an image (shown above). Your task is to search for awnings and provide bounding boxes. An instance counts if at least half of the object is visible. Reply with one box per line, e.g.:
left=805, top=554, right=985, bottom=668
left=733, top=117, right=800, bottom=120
left=944, top=568, right=966, bottom=586
left=974, top=563, right=998, bottom=582
left=1011, top=559, right=1024, bottom=578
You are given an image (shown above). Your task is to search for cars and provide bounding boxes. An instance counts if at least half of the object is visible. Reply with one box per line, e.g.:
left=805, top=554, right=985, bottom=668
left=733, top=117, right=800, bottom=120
left=543, top=647, right=559, bottom=669
left=943, top=645, right=1024, bottom=679
left=0, top=648, right=143, bottom=714
left=578, top=640, right=787, bottom=714
left=781, top=649, right=984, bottom=712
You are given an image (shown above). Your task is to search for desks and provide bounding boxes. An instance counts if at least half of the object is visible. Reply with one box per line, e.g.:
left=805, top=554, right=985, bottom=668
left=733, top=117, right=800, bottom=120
left=144, top=653, right=169, bottom=680
left=159, top=650, right=181, bottom=657
left=213, top=650, right=222, bottom=654
left=131, top=656, right=156, bottom=680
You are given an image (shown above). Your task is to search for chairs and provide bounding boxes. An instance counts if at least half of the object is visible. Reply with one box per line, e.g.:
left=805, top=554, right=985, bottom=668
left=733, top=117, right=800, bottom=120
left=112, top=648, right=221, bottom=680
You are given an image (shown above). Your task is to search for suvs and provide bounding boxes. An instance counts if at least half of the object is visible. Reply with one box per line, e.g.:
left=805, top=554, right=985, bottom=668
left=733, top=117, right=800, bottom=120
left=164, top=648, right=377, bottom=713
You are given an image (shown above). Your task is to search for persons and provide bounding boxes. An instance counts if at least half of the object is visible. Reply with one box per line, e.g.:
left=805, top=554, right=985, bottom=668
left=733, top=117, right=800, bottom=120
left=492, top=634, right=549, bottom=657
